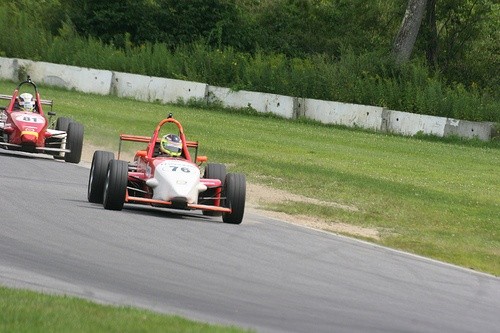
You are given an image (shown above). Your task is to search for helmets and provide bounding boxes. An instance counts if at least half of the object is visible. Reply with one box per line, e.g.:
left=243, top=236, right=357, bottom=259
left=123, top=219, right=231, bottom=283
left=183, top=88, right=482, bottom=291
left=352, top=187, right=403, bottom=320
left=158, top=134, right=182, bottom=157
left=17, top=93, right=36, bottom=112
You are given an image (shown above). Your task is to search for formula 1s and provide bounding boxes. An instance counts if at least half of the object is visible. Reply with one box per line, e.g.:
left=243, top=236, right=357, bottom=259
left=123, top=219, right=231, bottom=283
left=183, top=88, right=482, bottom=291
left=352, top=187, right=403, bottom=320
left=87, top=113, right=247, bottom=224
left=0, top=74, right=85, bottom=164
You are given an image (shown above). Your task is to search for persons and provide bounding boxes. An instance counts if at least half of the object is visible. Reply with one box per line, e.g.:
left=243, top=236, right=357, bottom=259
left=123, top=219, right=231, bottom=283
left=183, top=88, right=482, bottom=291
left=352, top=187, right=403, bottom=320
left=157, top=134, right=183, bottom=157
left=17, top=93, right=37, bottom=112
